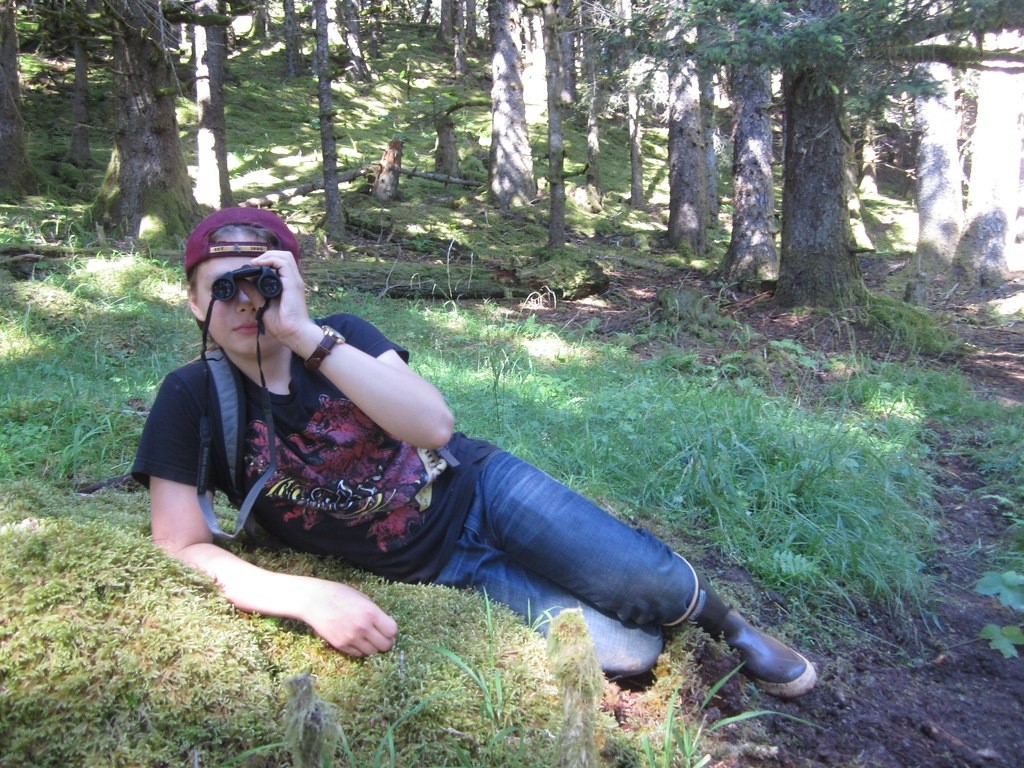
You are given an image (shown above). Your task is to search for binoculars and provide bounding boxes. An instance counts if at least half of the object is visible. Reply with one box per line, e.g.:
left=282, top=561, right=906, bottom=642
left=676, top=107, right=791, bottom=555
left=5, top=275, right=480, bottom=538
left=210, top=264, right=284, bottom=302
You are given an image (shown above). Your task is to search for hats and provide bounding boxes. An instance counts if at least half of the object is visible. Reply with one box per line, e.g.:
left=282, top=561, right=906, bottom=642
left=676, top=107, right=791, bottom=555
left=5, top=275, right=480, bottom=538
left=185, top=207, right=301, bottom=273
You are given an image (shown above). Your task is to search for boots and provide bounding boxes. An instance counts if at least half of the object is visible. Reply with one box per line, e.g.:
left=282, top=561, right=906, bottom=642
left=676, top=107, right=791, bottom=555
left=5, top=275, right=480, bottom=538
left=662, top=552, right=818, bottom=699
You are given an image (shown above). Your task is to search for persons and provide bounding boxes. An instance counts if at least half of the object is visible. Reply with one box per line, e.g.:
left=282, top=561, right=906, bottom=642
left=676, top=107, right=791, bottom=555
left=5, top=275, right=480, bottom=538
left=130, top=207, right=817, bottom=697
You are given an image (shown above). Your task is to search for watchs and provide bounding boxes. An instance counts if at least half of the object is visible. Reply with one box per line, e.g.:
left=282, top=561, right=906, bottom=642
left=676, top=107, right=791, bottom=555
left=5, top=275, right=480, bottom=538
left=303, top=325, right=345, bottom=372
